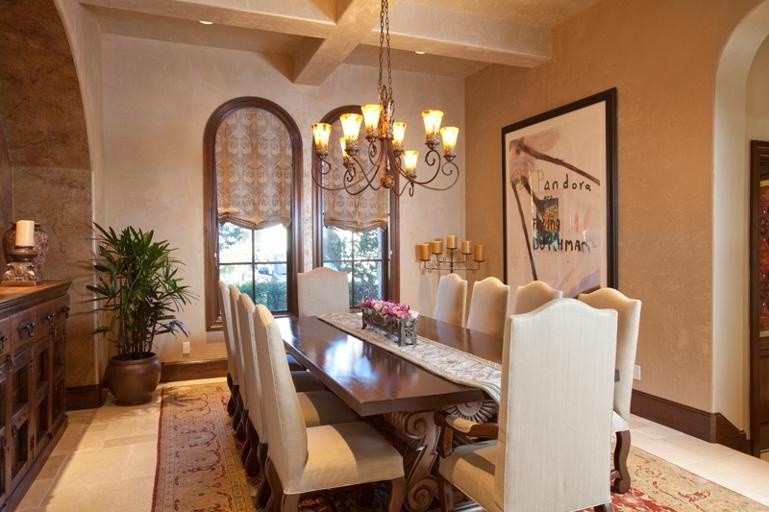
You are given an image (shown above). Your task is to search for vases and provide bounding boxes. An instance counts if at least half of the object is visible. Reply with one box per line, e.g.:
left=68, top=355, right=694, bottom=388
left=4, top=221, right=50, bottom=282
left=362, top=310, right=416, bottom=345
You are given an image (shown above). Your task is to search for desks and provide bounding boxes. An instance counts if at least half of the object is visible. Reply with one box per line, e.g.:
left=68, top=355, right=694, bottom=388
left=279, top=306, right=540, bottom=512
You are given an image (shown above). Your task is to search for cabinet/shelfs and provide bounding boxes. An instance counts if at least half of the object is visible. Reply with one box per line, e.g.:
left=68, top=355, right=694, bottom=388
left=0, top=278, right=73, bottom=510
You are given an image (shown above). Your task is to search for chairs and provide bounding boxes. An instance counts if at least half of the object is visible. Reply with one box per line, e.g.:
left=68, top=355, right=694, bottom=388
left=579, top=283, right=646, bottom=494
left=512, top=281, right=563, bottom=333
left=216, top=276, right=307, bottom=414
left=251, top=304, right=402, bottom=510
left=226, top=285, right=324, bottom=436
left=467, top=276, right=511, bottom=336
left=435, top=296, right=621, bottom=510
left=433, top=272, right=468, bottom=328
left=239, top=296, right=355, bottom=470
left=295, top=267, right=350, bottom=306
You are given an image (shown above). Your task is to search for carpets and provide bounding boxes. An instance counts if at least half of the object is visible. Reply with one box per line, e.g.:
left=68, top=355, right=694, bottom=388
left=150, top=377, right=769, bottom=511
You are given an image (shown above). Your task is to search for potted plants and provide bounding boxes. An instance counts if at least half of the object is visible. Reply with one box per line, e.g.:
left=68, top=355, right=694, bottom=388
left=77, top=216, right=197, bottom=406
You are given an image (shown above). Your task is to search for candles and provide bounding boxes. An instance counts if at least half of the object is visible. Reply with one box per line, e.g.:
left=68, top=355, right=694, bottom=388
left=418, top=235, right=485, bottom=262
left=17, top=220, right=36, bottom=246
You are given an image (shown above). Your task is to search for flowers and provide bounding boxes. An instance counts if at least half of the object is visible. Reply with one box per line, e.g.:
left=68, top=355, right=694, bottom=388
left=360, top=294, right=415, bottom=320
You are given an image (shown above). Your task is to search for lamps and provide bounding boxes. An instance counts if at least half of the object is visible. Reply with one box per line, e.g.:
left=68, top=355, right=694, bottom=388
left=311, top=1, right=463, bottom=199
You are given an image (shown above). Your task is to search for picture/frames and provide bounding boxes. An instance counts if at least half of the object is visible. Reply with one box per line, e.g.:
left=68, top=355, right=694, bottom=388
left=500, top=86, right=618, bottom=295
